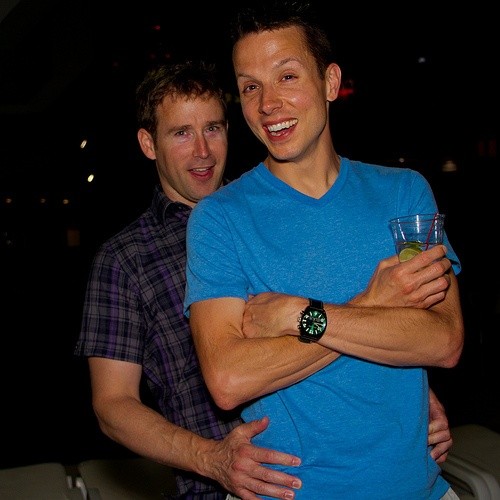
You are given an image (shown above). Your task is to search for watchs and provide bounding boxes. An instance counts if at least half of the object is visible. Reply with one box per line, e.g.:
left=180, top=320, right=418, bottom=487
left=297, top=298, right=327, bottom=344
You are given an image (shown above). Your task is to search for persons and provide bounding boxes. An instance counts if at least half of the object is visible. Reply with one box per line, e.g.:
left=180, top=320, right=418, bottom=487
left=73, top=60, right=454, bottom=499
left=182, top=6, right=464, bottom=500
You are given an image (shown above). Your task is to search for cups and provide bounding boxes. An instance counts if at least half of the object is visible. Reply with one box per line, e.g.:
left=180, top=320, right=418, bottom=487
left=389, top=214, right=444, bottom=270
